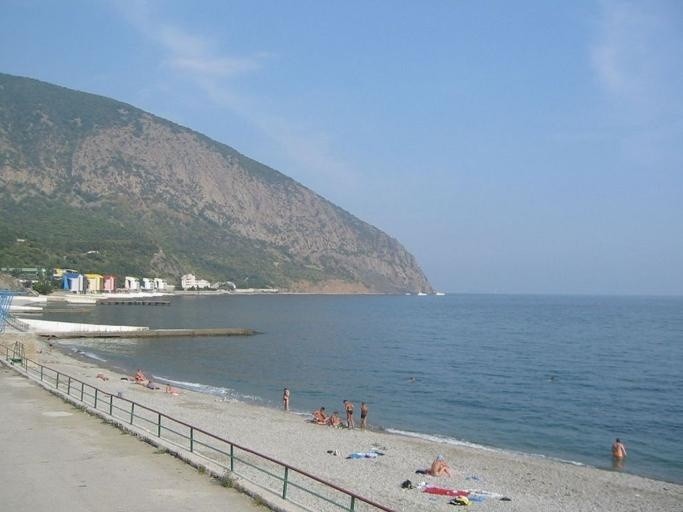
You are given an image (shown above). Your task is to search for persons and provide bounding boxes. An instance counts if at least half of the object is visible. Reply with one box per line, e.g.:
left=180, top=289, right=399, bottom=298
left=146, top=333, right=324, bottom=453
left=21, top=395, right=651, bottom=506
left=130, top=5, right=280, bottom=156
left=360, top=401, right=368, bottom=433
left=313, top=406, right=342, bottom=427
left=343, top=400, right=355, bottom=430
left=430, top=454, right=452, bottom=477
left=612, top=438, right=627, bottom=457
left=165, top=383, right=172, bottom=393
left=48, top=337, right=53, bottom=352
left=148, top=380, right=159, bottom=390
left=283, top=388, right=290, bottom=411
left=136, top=368, right=145, bottom=383
